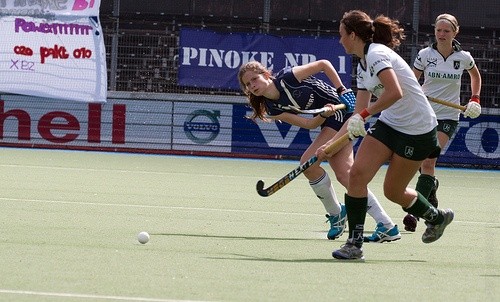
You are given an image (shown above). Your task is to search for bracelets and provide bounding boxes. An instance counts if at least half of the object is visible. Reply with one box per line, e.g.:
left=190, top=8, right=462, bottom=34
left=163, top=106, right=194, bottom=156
left=320, top=113, right=329, bottom=118
left=337, top=86, right=346, bottom=96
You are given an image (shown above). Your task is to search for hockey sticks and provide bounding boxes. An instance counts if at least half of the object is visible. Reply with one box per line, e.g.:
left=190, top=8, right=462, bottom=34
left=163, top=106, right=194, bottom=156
left=286, top=103, right=346, bottom=114
left=425, top=94, right=467, bottom=113
left=256, top=123, right=370, bottom=198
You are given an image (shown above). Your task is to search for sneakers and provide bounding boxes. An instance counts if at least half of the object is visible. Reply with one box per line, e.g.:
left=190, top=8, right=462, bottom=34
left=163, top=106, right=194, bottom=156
left=429, top=178, right=439, bottom=209
left=332, top=240, right=364, bottom=260
left=422, top=210, right=454, bottom=243
left=362, top=222, right=402, bottom=243
left=325, top=202, right=347, bottom=240
left=403, top=214, right=417, bottom=232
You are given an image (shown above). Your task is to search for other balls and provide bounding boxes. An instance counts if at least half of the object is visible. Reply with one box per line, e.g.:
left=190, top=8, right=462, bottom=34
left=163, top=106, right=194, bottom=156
left=137, top=231, right=150, bottom=246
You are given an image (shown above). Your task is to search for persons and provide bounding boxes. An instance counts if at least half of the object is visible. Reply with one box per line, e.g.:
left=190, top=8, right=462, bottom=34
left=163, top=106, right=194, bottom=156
left=236, top=59, right=401, bottom=243
left=402, top=13, right=482, bottom=232
left=314, top=10, right=454, bottom=261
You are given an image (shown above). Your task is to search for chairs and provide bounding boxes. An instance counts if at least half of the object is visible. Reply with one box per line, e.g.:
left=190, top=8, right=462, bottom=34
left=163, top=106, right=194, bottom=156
left=100, top=0, right=500, bottom=109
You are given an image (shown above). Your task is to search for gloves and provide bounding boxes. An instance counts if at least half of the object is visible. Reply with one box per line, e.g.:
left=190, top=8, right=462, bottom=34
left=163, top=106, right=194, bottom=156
left=461, top=95, right=482, bottom=118
left=346, top=107, right=371, bottom=141
left=338, top=88, right=357, bottom=113
